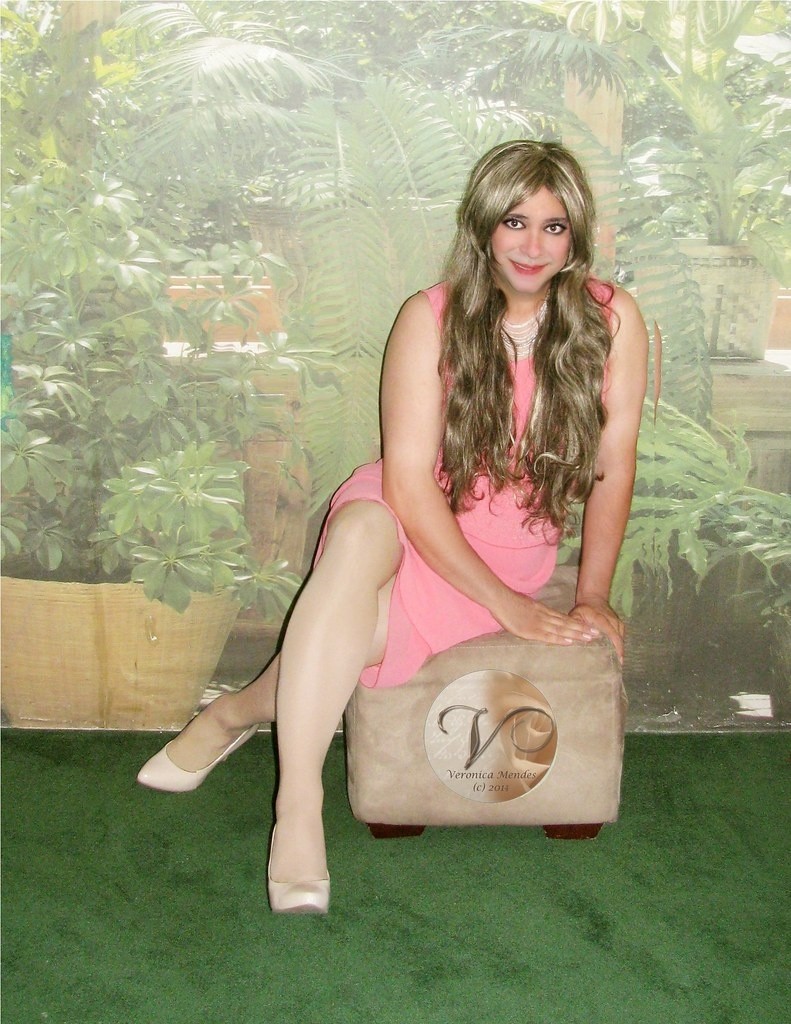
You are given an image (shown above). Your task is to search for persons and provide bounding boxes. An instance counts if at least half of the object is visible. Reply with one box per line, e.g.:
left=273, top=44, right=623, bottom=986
left=136, top=144, right=651, bottom=912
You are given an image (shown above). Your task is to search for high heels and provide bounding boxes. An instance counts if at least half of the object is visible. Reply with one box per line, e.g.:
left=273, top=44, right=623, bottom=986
left=268, top=819, right=331, bottom=914
left=135, top=722, right=261, bottom=792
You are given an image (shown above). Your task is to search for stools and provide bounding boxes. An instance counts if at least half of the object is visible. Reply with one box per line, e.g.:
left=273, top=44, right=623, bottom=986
left=344, top=567, right=623, bottom=838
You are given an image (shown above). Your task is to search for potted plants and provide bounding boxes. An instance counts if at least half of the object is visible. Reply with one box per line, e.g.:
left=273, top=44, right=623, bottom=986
left=627, top=67, right=790, bottom=363
left=1, top=128, right=307, bottom=732
